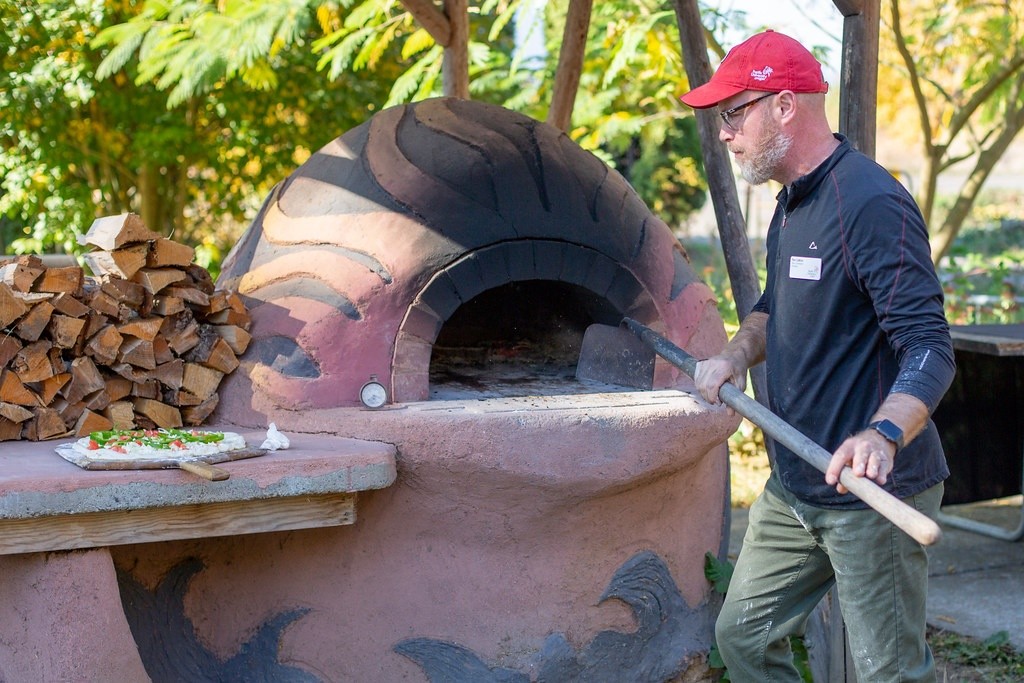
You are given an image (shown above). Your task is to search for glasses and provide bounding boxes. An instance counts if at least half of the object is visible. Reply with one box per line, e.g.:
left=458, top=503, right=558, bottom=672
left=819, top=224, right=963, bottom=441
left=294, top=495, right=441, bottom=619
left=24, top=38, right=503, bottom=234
left=719, top=92, right=779, bottom=129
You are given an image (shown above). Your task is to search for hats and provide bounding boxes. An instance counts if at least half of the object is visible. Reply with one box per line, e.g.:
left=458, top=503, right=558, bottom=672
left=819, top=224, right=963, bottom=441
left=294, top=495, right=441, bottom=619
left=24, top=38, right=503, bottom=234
left=680, top=29, right=829, bottom=110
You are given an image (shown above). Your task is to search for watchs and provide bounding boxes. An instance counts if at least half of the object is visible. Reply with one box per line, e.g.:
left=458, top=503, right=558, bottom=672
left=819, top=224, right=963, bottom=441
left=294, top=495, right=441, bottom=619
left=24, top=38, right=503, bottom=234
left=864, top=418, right=903, bottom=458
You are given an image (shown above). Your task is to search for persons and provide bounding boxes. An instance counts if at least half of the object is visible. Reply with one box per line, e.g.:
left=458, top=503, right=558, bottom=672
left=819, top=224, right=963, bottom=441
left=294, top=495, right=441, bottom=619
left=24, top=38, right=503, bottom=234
left=695, top=28, right=956, bottom=683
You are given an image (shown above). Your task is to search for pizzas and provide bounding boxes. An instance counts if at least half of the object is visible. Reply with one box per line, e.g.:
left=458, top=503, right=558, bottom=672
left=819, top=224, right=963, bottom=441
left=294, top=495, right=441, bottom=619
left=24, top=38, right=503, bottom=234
left=72, top=430, right=244, bottom=457
